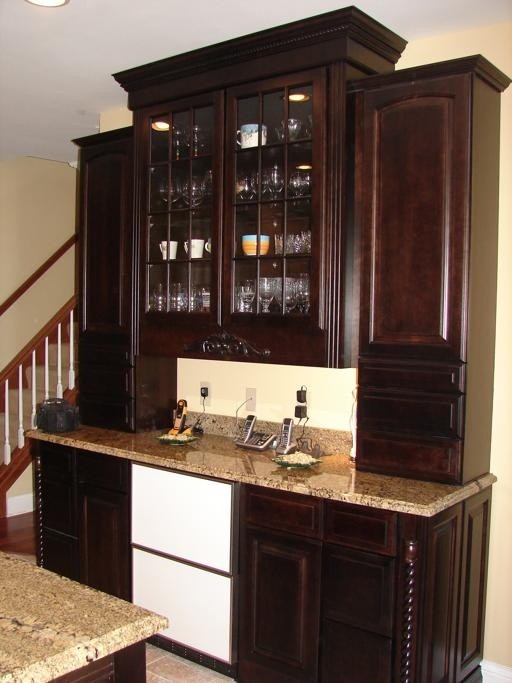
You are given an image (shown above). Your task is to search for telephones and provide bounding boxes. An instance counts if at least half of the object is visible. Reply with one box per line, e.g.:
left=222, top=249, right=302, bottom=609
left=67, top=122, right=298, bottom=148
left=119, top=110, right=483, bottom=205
left=277, top=418, right=297, bottom=455
left=168, top=400, right=193, bottom=437
left=234, top=415, right=276, bottom=451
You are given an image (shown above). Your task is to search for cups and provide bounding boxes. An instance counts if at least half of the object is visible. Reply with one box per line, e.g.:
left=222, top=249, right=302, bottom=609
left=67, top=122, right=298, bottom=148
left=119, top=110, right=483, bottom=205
left=274, top=230, right=311, bottom=255
left=150, top=281, right=210, bottom=312
left=274, top=118, right=303, bottom=144
left=158, top=167, right=311, bottom=215
left=273, top=272, right=312, bottom=313
left=159, top=240, right=178, bottom=260
left=237, top=123, right=268, bottom=149
left=184, top=237, right=211, bottom=258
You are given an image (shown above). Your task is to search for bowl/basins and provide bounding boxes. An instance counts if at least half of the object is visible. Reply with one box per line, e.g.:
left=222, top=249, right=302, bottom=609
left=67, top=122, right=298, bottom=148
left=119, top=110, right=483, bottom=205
left=241, top=234, right=270, bottom=256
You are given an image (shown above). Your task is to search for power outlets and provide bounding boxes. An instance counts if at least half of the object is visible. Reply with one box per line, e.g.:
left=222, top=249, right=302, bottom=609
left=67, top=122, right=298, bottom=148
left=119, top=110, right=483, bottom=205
left=198, top=383, right=211, bottom=407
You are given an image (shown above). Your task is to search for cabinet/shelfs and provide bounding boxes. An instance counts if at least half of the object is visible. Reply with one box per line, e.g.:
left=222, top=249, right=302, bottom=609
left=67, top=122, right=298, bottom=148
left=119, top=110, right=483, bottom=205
left=129, top=461, right=235, bottom=680
left=320, top=489, right=496, bottom=683
left=234, top=488, right=317, bottom=683
left=27, top=442, right=77, bottom=585
left=342, top=68, right=501, bottom=488
left=74, top=451, right=132, bottom=603
left=128, top=60, right=352, bottom=371
left=68, top=126, right=176, bottom=432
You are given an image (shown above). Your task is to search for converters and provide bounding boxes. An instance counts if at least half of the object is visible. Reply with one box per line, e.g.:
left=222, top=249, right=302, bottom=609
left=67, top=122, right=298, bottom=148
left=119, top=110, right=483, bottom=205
left=201, top=388, right=208, bottom=397
left=297, top=391, right=306, bottom=402
left=295, top=405, right=307, bottom=418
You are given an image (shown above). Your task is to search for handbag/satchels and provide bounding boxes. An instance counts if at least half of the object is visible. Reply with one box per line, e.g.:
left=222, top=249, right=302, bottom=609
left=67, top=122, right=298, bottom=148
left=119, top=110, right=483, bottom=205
left=34, top=397, right=79, bottom=434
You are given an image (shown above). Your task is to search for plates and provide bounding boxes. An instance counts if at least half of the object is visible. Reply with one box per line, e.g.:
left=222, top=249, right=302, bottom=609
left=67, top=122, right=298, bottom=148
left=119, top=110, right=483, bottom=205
left=159, top=433, right=198, bottom=446
left=271, top=454, right=323, bottom=468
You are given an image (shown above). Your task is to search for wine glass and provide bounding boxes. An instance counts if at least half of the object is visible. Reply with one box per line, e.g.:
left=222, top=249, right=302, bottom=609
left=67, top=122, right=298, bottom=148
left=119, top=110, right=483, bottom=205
left=237, top=279, right=257, bottom=313
left=254, top=276, right=279, bottom=312
